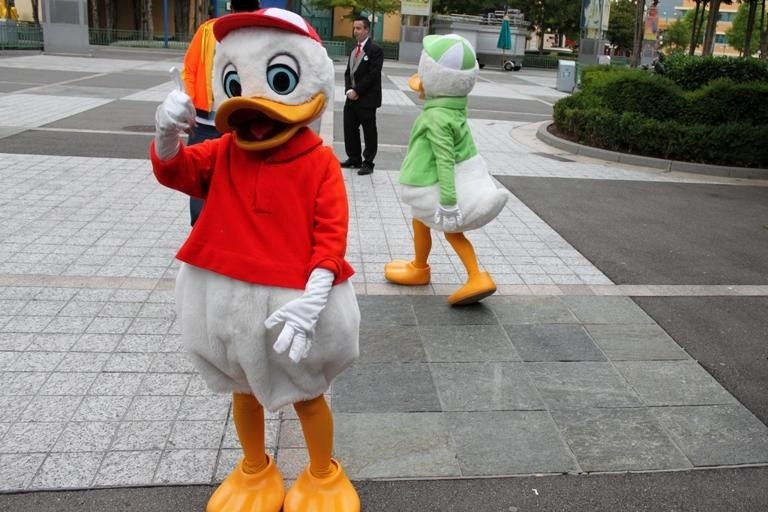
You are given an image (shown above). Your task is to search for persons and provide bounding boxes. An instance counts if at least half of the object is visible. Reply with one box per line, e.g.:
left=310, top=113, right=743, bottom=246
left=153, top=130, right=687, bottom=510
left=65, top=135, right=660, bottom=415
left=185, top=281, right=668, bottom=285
left=340, top=17, right=384, bottom=175
left=181, top=0, right=260, bottom=227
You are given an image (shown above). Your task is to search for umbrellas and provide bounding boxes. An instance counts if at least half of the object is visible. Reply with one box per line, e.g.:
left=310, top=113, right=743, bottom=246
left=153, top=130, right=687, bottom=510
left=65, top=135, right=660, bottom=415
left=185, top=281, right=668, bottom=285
left=496, top=14, right=512, bottom=70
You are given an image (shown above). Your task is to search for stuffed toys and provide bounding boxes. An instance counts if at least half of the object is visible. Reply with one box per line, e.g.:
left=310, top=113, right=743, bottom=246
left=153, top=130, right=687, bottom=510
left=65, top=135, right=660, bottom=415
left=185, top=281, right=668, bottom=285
left=150, top=8, right=361, bottom=512
left=383, top=34, right=509, bottom=305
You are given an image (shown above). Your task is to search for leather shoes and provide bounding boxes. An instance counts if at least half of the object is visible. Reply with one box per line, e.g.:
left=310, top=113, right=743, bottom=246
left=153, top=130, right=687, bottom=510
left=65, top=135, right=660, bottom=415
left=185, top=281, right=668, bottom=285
left=340, top=159, right=362, bottom=168
left=358, top=162, right=373, bottom=175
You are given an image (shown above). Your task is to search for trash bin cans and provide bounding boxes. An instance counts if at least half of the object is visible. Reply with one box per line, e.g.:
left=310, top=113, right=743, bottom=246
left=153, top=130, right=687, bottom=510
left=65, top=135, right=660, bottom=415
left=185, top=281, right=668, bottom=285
left=598, top=55, right=626, bottom=66
left=557, top=60, right=576, bottom=93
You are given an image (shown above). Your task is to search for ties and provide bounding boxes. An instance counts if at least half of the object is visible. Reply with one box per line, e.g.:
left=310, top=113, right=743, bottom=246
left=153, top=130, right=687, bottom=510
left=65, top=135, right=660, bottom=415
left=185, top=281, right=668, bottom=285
left=355, top=44, right=361, bottom=58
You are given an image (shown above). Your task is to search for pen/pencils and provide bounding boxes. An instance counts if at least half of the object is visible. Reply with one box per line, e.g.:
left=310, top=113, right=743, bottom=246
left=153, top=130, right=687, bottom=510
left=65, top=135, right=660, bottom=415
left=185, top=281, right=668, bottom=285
left=170, top=67, right=184, bottom=93
